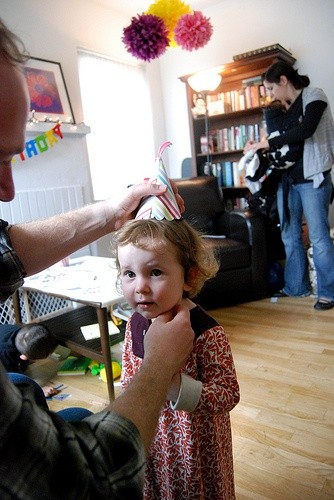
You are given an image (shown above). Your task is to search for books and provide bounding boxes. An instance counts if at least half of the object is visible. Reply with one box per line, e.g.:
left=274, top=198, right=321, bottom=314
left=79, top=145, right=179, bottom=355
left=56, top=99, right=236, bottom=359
left=209, top=123, right=259, bottom=152
left=81, top=320, right=120, bottom=341
left=208, top=85, right=259, bottom=116
left=112, top=303, right=135, bottom=321
left=58, top=358, right=90, bottom=376
left=211, top=161, right=246, bottom=187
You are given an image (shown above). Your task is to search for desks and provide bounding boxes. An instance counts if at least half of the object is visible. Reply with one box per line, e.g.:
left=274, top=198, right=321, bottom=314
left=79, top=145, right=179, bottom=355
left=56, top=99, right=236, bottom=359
left=15, top=256, right=125, bottom=406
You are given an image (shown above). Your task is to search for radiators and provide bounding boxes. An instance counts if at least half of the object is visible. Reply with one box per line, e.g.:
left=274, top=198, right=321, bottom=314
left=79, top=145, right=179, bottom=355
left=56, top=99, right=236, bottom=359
left=0, top=185, right=98, bottom=326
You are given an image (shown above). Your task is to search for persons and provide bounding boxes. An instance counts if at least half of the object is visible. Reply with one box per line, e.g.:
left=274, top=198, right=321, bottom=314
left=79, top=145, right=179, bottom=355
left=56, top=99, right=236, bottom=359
left=111, top=216, right=241, bottom=500
left=0, top=18, right=195, bottom=500
left=243, top=61, right=334, bottom=310
left=0, top=323, right=58, bottom=397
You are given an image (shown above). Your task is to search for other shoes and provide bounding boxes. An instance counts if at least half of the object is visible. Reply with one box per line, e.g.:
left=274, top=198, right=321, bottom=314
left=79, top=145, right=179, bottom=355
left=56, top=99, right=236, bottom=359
left=314, top=298, right=334, bottom=310
left=272, top=290, right=287, bottom=297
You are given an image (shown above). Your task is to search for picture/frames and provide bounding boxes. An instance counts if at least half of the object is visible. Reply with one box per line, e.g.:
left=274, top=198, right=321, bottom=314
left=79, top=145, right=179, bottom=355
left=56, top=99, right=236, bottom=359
left=23, top=55, right=76, bottom=124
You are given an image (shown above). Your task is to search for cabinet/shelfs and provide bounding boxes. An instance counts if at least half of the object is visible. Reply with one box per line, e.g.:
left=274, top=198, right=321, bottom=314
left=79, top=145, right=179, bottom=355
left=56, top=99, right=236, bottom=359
left=177, top=50, right=296, bottom=209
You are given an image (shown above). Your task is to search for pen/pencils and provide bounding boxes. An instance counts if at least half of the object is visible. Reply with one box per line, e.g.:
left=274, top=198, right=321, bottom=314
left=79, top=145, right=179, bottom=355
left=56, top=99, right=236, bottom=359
left=55, top=383, right=63, bottom=389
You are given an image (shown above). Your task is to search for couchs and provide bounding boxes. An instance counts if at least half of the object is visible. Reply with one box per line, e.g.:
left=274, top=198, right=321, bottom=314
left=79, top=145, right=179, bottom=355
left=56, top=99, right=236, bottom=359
left=172, top=176, right=272, bottom=310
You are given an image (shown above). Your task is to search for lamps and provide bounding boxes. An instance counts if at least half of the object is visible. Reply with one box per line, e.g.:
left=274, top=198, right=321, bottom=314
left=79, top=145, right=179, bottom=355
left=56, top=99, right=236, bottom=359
left=187, top=73, right=222, bottom=176
